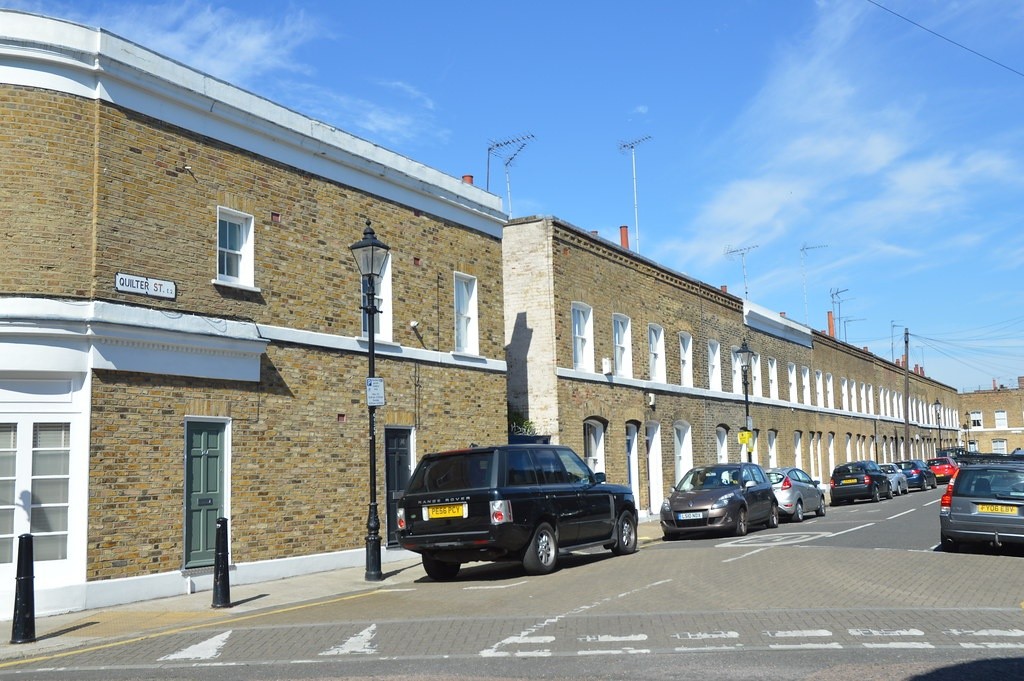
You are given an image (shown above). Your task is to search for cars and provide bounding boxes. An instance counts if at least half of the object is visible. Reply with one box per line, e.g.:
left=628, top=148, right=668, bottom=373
left=827, top=461, right=893, bottom=504
left=898, top=460, right=938, bottom=490
left=660, top=462, right=779, bottom=537
left=928, top=458, right=957, bottom=482
left=878, top=463, right=909, bottom=496
left=763, top=468, right=827, bottom=522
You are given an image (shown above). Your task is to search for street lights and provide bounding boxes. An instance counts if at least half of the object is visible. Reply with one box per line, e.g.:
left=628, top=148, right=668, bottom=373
left=934, top=399, right=944, bottom=453
left=964, top=412, right=971, bottom=450
left=735, top=337, right=756, bottom=463
left=348, top=216, right=391, bottom=581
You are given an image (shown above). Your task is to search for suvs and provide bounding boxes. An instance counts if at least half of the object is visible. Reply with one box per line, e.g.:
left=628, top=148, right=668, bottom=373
left=939, top=464, right=1024, bottom=550
left=395, top=444, right=638, bottom=581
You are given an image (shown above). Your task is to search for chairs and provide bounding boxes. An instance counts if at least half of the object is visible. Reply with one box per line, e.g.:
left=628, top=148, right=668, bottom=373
left=898, top=465, right=902, bottom=470
left=730, top=472, right=745, bottom=485
left=974, top=478, right=991, bottom=494
left=840, top=468, right=851, bottom=474
